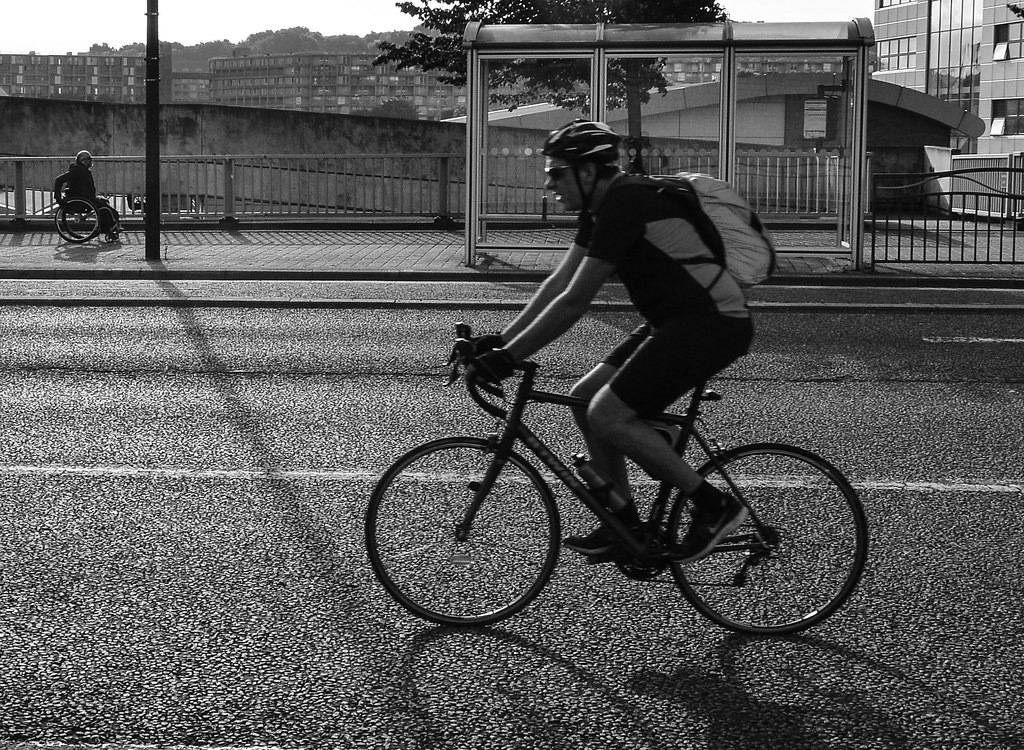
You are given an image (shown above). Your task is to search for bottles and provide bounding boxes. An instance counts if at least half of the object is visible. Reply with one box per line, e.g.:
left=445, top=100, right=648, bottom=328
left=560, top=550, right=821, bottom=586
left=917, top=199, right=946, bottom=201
left=572, top=452, right=629, bottom=509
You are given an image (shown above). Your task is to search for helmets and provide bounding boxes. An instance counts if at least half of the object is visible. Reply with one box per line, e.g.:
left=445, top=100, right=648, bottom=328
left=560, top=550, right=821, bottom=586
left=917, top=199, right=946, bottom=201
left=541, top=118, right=621, bottom=164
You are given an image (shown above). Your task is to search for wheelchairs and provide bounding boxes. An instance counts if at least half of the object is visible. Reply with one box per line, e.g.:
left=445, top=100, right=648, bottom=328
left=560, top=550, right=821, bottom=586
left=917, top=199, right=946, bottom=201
left=54, top=185, right=123, bottom=243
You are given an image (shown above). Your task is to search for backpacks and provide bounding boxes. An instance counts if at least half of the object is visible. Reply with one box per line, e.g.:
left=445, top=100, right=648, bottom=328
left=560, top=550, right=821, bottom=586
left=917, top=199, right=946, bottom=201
left=588, top=171, right=777, bottom=287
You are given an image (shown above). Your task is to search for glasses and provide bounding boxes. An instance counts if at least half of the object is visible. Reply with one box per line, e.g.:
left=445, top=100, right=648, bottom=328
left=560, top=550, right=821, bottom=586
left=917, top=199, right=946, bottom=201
left=545, top=165, right=574, bottom=182
left=81, top=157, right=92, bottom=161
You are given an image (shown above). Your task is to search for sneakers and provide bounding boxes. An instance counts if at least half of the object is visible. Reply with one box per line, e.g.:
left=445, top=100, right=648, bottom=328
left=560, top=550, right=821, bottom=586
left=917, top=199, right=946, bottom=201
left=672, top=493, right=748, bottom=563
left=564, top=513, right=639, bottom=554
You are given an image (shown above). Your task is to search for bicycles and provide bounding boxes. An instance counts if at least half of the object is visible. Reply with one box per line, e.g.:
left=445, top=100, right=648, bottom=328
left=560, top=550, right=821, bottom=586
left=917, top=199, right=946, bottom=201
left=362, top=321, right=870, bottom=637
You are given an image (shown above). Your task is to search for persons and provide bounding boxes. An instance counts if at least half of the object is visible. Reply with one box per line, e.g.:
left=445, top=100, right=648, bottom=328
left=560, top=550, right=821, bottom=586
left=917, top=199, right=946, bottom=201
left=54, top=150, right=124, bottom=240
left=456, top=119, right=752, bottom=563
